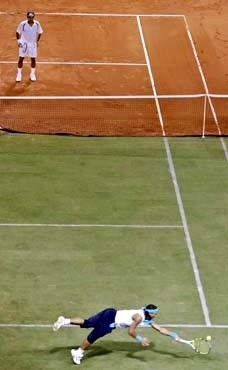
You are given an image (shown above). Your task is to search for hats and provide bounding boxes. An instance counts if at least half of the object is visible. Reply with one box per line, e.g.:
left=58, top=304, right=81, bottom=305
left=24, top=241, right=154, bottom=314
left=28, top=12, right=35, bottom=17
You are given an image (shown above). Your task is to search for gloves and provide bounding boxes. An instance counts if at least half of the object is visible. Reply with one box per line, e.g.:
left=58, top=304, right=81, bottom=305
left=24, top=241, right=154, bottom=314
left=17, top=39, right=24, bottom=47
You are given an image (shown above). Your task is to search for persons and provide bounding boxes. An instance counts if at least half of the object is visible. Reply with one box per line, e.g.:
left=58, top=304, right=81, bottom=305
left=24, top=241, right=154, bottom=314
left=52, top=304, right=179, bottom=365
left=16, top=11, right=43, bottom=82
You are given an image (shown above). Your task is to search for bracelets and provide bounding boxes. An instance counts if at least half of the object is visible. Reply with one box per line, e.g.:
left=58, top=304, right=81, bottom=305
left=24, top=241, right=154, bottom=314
left=135, top=335, right=142, bottom=342
left=170, top=332, right=177, bottom=339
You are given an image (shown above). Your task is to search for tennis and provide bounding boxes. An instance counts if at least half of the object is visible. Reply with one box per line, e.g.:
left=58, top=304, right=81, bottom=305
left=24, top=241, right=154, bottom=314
left=206, top=335, right=211, bottom=342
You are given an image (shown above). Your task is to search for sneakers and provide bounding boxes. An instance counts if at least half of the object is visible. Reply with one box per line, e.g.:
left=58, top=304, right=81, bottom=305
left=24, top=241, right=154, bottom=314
left=16, top=72, right=22, bottom=81
left=52, top=316, right=65, bottom=331
left=71, top=349, right=83, bottom=365
left=29, top=74, right=36, bottom=80
